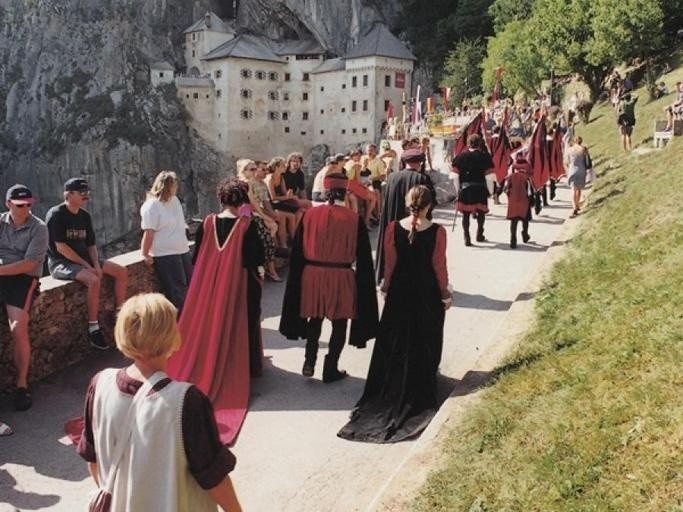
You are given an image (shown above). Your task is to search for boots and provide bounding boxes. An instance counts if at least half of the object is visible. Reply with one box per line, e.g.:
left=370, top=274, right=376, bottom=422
left=509, top=234, right=516, bottom=248
left=521, top=231, right=530, bottom=243
left=543, top=199, right=548, bottom=206
left=302, top=344, right=318, bottom=376
left=475, top=228, right=484, bottom=241
left=550, top=192, right=554, bottom=199
left=534, top=206, right=541, bottom=215
left=493, top=189, right=501, bottom=201
left=463, top=232, right=471, bottom=246
left=323, top=355, right=346, bottom=382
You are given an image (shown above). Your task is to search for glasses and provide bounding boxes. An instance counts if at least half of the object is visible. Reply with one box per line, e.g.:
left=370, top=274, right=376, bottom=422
left=330, top=162, right=337, bottom=164
left=13, top=204, right=31, bottom=207
left=74, top=191, right=90, bottom=195
left=246, top=168, right=256, bottom=171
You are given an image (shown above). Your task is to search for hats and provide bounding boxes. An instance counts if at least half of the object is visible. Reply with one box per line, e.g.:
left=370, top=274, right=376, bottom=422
left=621, top=93, right=630, bottom=100
left=236, top=159, right=254, bottom=180
left=324, top=157, right=337, bottom=165
left=335, top=153, right=350, bottom=161
left=401, top=149, right=424, bottom=162
left=381, top=141, right=390, bottom=148
left=6, top=183, right=36, bottom=205
left=66, top=178, right=92, bottom=192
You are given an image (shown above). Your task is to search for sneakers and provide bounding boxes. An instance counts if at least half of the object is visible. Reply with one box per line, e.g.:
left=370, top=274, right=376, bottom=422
left=572, top=208, right=576, bottom=216
left=274, top=247, right=288, bottom=258
left=86, top=328, right=109, bottom=350
left=576, top=205, right=580, bottom=210
left=15, top=384, right=32, bottom=411
left=365, top=222, right=371, bottom=231
left=369, top=213, right=377, bottom=221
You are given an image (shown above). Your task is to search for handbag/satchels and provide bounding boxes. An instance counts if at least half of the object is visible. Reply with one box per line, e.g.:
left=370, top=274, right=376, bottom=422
left=89, top=491, right=111, bottom=512
left=585, top=154, right=591, bottom=168
left=272, top=200, right=300, bottom=212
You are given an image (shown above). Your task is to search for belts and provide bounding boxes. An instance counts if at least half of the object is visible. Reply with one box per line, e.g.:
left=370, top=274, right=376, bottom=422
left=304, top=260, right=352, bottom=268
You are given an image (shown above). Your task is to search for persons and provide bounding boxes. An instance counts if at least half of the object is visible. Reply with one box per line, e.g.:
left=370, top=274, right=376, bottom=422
left=610, top=75, right=683, bottom=151
left=0, top=420, right=14, bottom=436
left=64, top=178, right=266, bottom=449
left=378, top=83, right=436, bottom=177
left=253, top=161, right=291, bottom=258
left=312, top=141, right=378, bottom=232
left=336, top=186, right=452, bottom=443
left=278, top=172, right=380, bottom=382
left=141, top=169, right=194, bottom=322
left=263, top=157, right=303, bottom=241
left=236, top=159, right=282, bottom=282
left=76, top=292, right=245, bottom=512
left=449, top=94, right=595, bottom=249
left=282, top=152, right=308, bottom=228
left=45, top=177, right=128, bottom=350
left=374, top=149, right=438, bottom=287
left=0, top=183, right=50, bottom=412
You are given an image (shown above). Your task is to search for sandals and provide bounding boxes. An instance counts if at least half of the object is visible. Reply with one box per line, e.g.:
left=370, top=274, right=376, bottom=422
left=0, top=422, right=13, bottom=436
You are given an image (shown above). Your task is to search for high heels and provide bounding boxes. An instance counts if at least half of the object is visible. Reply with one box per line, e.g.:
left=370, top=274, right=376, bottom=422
left=266, top=270, right=282, bottom=282
